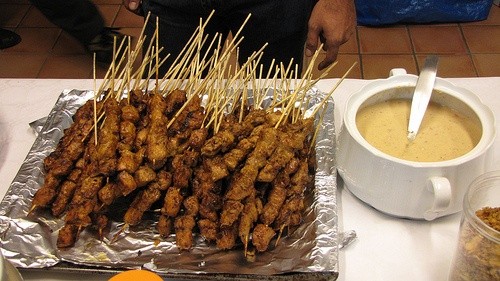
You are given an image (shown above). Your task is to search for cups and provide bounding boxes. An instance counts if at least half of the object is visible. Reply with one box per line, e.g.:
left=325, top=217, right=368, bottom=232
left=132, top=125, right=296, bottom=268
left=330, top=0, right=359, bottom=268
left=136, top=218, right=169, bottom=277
left=448, top=171, right=500, bottom=281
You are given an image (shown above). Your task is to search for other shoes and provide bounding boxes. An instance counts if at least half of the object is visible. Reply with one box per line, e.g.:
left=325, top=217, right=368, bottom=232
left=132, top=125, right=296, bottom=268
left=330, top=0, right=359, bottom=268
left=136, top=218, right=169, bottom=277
left=89, top=26, right=132, bottom=62
left=0, top=28, right=22, bottom=49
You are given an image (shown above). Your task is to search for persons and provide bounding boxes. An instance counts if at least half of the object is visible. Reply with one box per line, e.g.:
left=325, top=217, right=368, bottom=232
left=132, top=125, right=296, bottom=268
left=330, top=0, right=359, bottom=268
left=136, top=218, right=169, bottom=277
left=124, top=0, right=358, bottom=79
left=0, top=0, right=135, bottom=71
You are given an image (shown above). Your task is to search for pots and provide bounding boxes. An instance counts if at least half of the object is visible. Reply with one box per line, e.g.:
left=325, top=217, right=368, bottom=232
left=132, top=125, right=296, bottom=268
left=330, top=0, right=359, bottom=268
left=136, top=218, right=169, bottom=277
left=336, top=67, right=497, bottom=221
